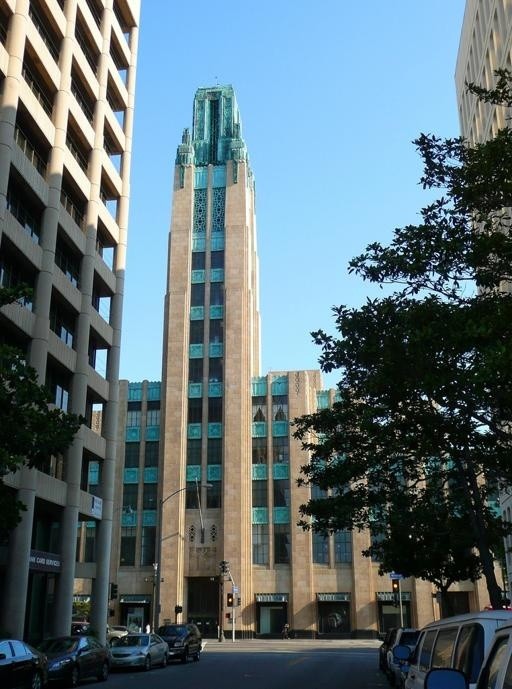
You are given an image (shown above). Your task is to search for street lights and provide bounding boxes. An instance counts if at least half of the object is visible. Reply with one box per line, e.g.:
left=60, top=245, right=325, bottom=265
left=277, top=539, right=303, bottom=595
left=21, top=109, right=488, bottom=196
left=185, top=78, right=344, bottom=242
left=154, top=483, right=213, bottom=633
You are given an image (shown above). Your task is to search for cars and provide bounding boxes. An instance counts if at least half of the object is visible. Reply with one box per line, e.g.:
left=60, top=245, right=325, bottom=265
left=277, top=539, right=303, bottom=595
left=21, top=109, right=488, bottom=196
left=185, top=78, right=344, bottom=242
left=0, top=639, right=49, bottom=689
left=155, top=623, right=202, bottom=664
left=70, top=621, right=138, bottom=645
left=37, top=635, right=112, bottom=688
left=378, top=609, right=511, bottom=688
left=108, top=632, right=170, bottom=672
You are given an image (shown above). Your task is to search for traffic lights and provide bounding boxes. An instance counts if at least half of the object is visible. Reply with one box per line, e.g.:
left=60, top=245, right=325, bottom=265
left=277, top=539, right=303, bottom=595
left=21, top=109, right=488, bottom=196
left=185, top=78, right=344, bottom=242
left=392, top=578, right=400, bottom=594
left=227, top=592, right=234, bottom=607
